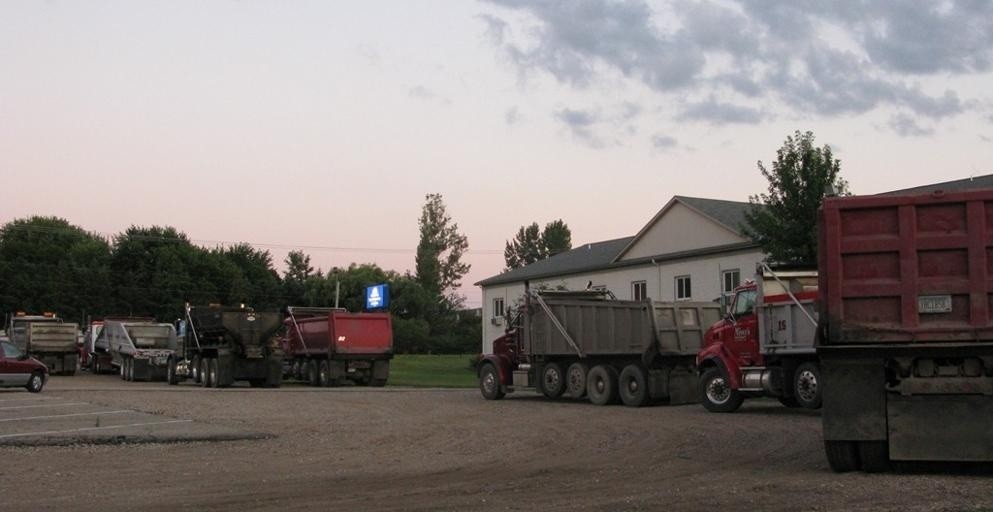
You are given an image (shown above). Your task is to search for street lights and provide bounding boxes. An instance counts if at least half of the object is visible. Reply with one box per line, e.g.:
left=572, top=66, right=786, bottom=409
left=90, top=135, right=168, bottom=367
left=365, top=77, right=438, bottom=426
left=333, top=267, right=340, bottom=308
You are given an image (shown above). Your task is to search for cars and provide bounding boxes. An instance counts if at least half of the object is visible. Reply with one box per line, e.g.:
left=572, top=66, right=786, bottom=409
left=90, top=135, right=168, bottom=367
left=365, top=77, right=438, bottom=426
left=0, top=340, right=50, bottom=393
